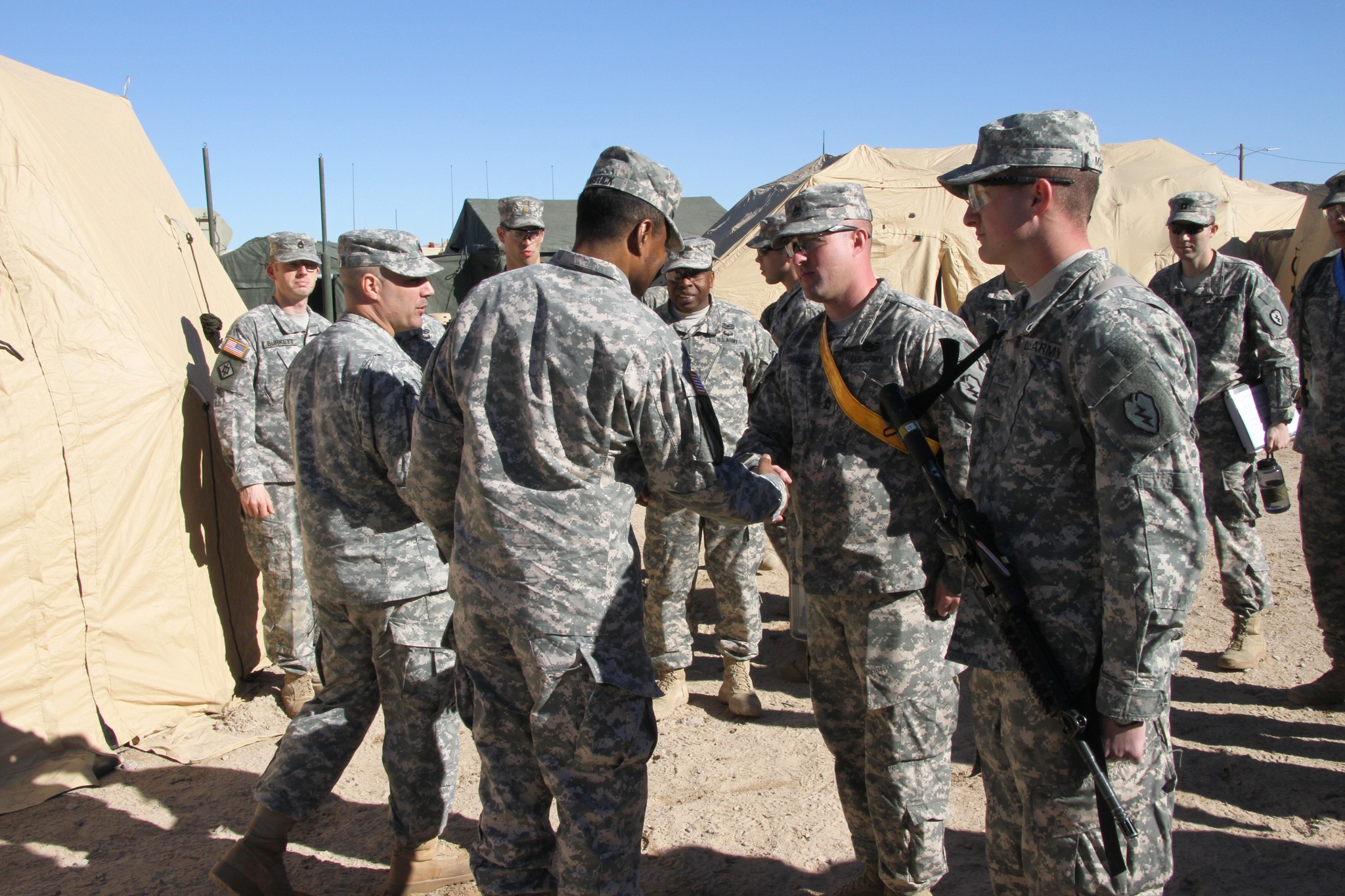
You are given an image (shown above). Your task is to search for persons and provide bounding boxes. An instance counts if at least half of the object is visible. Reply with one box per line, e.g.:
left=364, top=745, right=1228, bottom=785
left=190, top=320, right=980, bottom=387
left=496, top=198, right=545, bottom=273
left=744, top=212, right=829, bottom=685
left=406, top=146, right=787, bottom=896
left=1287, top=169, right=1345, bottom=707
left=957, top=264, right=1025, bottom=356
left=731, top=183, right=988, bottom=896
left=210, top=229, right=477, bottom=896
left=1147, top=191, right=1302, bottom=669
left=946, top=109, right=1207, bottom=896
left=642, top=238, right=778, bottom=722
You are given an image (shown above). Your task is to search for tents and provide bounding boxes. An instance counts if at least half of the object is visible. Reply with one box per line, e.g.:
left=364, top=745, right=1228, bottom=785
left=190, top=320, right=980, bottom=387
left=0, top=54, right=278, bottom=814
left=220, top=139, right=1337, bottom=318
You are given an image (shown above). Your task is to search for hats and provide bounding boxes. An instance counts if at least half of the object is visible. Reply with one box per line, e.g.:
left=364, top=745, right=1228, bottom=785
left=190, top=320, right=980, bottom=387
left=268, top=231, right=322, bottom=264
left=1165, top=191, right=1218, bottom=226
left=745, top=211, right=787, bottom=248
left=661, top=236, right=715, bottom=274
left=1318, top=169, right=1345, bottom=209
left=937, top=110, right=1103, bottom=201
left=583, top=146, right=685, bottom=251
left=497, top=196, right=545, bottom=229
left=337, top=229, right=444, bottom=278
left=770, top=183, right=874, bottom=250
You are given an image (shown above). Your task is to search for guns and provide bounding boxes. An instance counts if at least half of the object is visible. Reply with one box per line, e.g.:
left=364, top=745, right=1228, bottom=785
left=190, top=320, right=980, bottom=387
left=874, top=385, right=1139, bottom=840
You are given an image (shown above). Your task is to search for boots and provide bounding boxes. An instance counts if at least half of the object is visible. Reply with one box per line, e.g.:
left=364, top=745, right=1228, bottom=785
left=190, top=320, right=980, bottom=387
left=209, top=802, right=309, bottom=896
left=651, top=667, right=689, bottom=721
left=719, top=656, right=762, bottom=716
left=1286, top=656, right=1345, bottom=705
left=798, top=860, right=886, bottom=896
left=311, top=666, right=324, bottom=694
left=387, top=835, right=476, bottom=895
left=281, top=672, right=315, bottom=719
left=1218, top=615, right=1267, bottom=669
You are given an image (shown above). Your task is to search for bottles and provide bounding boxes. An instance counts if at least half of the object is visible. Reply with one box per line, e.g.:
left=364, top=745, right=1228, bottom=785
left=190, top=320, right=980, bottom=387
left=1256, top=452, right=1291, bottom=514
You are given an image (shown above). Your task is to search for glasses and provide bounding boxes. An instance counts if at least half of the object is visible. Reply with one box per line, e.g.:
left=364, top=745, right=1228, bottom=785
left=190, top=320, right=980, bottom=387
left=665, top=266, right=712, bottom=283
left=1170, top=222, right=1214, bottom=236
left=781, top=225, right=871, bottom=257
left=498, top=225, right=545, bottom=242
left=1322, top=204, right=1345, bottom=219
left=963, top=176, right=1075, bottom=215
left=757, top=247, right=773, bottom=257
left=272, top=259, right=317, bottom=271
